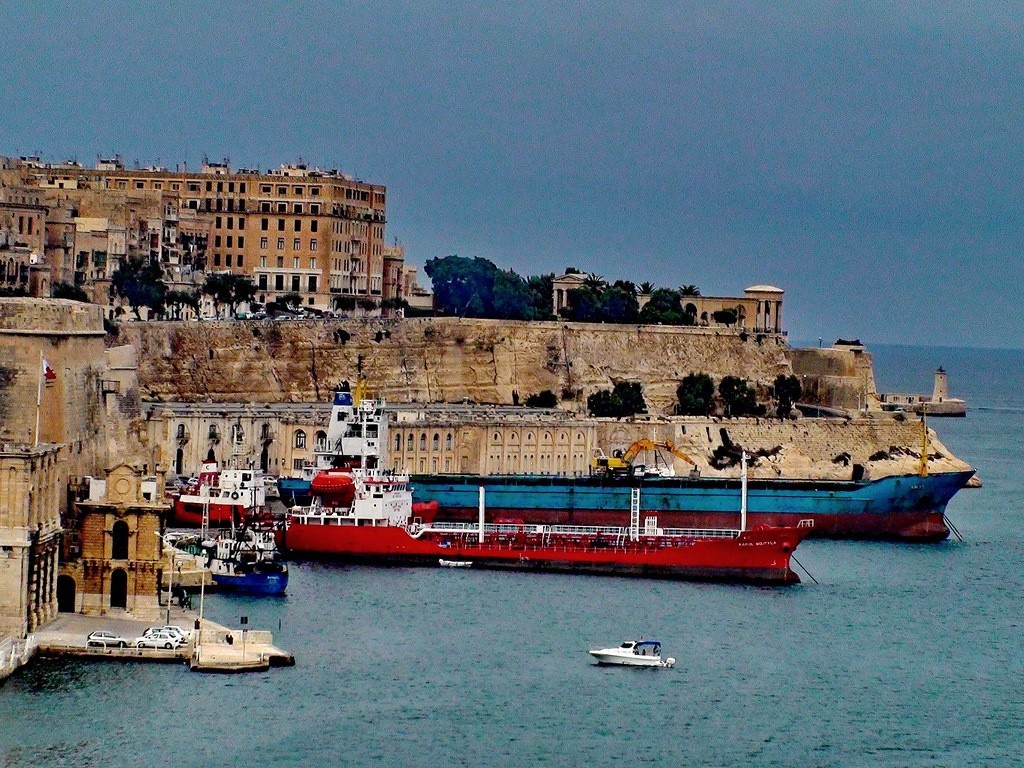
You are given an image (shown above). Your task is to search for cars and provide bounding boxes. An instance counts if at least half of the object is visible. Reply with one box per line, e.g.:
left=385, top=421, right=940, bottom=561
left=87, top=630, right=132, bottom=648
left=133, top=625, right=190, bottom=649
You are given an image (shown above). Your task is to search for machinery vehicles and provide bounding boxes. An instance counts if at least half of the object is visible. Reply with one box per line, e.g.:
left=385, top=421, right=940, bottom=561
left=589, top=438, right=703, bottom=482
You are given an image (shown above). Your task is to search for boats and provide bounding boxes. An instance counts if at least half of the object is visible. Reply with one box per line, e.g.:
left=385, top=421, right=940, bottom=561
left=165, top=382, right=979, bottom=593
left=588, top=639, right=676, bottom=668
